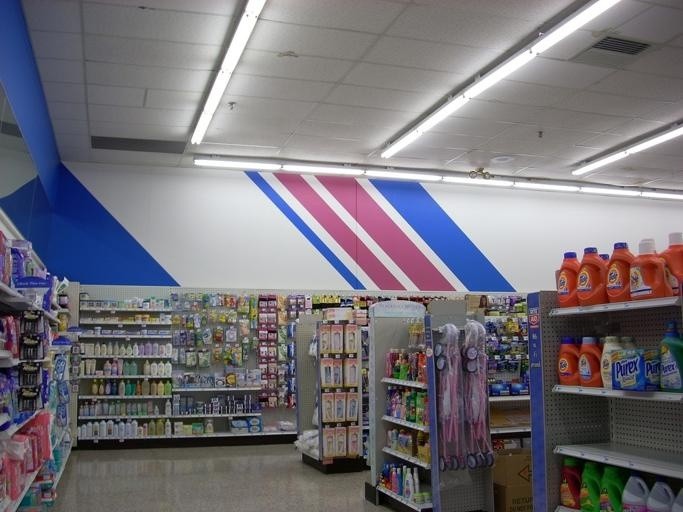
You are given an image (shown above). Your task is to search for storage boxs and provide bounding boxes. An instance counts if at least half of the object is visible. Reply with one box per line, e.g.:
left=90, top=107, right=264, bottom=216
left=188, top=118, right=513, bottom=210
left=488, top=449, right=534, bottom=512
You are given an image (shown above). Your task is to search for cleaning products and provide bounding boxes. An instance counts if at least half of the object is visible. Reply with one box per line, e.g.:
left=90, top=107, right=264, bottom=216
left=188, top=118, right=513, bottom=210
left=553, top=232, right=683, bottom=391
left=560, top=458, right=683, bottom=512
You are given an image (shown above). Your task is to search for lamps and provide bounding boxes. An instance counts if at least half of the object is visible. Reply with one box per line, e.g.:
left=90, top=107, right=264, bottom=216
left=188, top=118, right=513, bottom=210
left=564, top=117, right=683, bottom=181
left=186, top=0, right=277, bottom=146
left=375, top=0, right=634, bottom=158
left=183, top=148, right=683, bottom=210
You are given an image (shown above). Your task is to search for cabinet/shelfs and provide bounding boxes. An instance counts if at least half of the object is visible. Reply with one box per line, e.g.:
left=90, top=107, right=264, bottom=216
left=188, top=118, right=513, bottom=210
left=80, top=299, right=173, bottom=442
left=519, top=284, right=683, bottom=512
left=285, top=307, right=370, bottom=475
left=0, top=278, right=79, bottom=512
left=166, top=385, right=261, bottom=440
left=354, top=294, right=533, bottom=511
